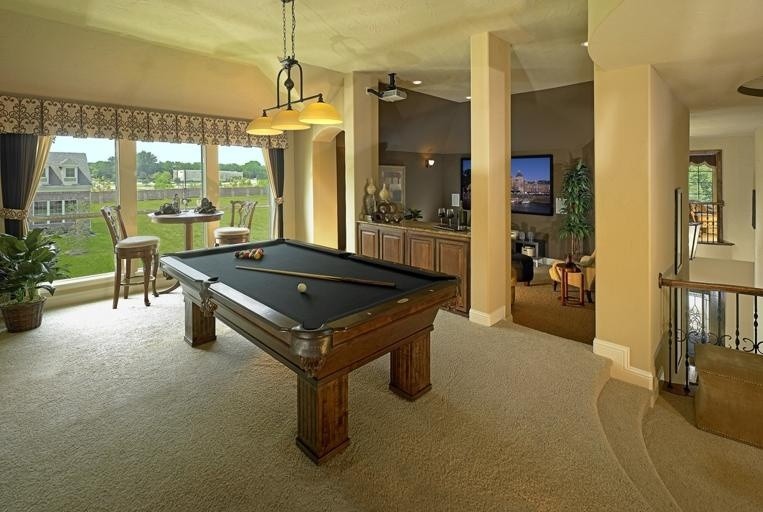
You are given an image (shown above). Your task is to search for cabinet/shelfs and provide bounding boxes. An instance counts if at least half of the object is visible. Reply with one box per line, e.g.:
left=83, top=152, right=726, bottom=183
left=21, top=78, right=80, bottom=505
left=405, top=229, right=470, bottom=313
left=358, top=222, right=405, bottom=264
left=511, top=239, right=545, bottom=258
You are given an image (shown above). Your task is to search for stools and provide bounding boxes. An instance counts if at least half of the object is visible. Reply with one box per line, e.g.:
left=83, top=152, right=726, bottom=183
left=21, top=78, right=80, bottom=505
left=512, top=253, right=533, bottom=286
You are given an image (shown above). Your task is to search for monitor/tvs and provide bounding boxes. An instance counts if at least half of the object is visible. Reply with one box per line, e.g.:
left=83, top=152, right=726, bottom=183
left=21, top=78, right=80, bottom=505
left=460, top=154, right=553, bottom=216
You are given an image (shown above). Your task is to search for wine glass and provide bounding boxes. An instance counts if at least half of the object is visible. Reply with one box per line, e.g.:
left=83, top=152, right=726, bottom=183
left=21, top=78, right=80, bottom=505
left=446, top=209, right=454, bottom=227
left=438, top=207, right=445, bottom=227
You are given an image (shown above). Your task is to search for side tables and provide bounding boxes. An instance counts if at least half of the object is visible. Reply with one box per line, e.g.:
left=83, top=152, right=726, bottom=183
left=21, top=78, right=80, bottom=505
left=555, top=263, right=585, bottom=307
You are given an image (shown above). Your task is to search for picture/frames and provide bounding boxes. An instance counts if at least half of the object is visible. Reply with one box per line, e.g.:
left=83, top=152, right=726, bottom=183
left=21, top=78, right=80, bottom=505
left=379, top=166, right=405, bottom=214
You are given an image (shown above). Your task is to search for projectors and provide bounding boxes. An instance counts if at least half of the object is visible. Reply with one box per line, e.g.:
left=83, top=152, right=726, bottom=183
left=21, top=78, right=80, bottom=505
left=367, top=88, right=407, bottom=103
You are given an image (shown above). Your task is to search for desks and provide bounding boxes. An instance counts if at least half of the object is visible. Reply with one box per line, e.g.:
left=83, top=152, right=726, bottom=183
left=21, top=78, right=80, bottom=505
left=159, top=238, right=460, bottom=467
left=151, top=209, right=224, bottom=294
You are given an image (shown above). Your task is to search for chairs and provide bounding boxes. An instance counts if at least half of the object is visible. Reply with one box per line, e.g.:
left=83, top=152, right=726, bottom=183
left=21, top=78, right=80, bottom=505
left=101, top=204, right=160, bottom=309
left=549, top=249, right=596, bottom=304
left=214, top=200, right=258, bottom=248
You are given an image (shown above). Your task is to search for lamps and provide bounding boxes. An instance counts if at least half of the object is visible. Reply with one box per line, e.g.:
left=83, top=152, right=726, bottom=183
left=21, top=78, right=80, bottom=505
left=425, top=159, right=434, bottom=168
left=688, top=222, right=702, bottom=260
left=246, top=0, right=344, bottom=136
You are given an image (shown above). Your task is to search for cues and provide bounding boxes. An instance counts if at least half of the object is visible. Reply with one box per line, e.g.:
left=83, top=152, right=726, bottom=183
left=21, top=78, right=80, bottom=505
left=234, top=266, right=396, bottom=288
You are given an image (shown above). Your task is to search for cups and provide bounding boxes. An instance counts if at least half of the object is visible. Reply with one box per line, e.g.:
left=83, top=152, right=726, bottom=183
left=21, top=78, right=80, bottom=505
left=457, top=211, right=467, bottom=231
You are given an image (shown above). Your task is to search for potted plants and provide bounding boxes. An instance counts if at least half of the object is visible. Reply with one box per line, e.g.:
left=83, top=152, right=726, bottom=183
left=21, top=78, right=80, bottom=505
left=0, top=227, right=71, bottom=332
left=557, top=156, right=594, bottom=263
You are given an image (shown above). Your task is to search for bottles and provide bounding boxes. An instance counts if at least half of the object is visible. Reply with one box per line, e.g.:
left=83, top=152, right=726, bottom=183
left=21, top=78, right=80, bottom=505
left=173, top=194, right=180, bottom=212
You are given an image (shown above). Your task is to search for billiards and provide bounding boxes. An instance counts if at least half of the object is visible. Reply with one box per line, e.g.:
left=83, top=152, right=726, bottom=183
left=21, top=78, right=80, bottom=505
left=297, top=282, right=307, bottom=293
left=235, top=248, right=264, bottom=259
